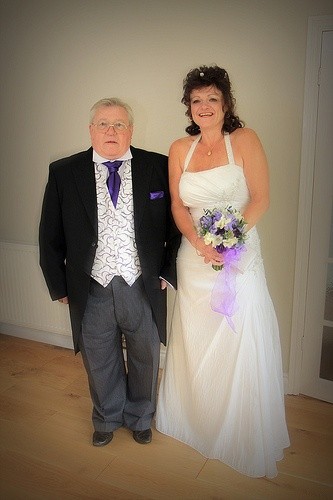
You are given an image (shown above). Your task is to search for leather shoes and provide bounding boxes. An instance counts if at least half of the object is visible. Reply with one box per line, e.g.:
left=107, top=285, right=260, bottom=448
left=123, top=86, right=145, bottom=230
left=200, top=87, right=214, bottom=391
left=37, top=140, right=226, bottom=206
left=133, top=428, right=152, bottom=444
left=93, top=431, right=113, bottom=447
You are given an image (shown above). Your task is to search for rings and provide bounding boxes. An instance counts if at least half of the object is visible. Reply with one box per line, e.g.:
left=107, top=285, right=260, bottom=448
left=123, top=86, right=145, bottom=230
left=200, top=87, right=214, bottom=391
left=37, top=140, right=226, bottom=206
left=213, top=255, right=217, bottom=259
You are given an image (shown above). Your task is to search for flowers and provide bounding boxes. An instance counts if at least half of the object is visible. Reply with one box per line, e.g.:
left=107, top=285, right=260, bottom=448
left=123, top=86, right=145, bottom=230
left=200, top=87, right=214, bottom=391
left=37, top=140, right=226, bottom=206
left=198, top=200, right=248, bottom=272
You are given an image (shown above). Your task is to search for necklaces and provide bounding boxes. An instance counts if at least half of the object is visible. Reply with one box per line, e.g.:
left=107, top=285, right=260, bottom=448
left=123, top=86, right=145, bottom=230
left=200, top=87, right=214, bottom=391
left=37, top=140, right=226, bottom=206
left=202, top=137, right=221, bottom=156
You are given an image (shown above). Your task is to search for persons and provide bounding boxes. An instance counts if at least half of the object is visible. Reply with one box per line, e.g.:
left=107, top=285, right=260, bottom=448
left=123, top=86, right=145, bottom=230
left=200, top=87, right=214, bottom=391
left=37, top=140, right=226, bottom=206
left=39, top=97, right=182, bottom=447
left=155, top=64, right=291, bottom=479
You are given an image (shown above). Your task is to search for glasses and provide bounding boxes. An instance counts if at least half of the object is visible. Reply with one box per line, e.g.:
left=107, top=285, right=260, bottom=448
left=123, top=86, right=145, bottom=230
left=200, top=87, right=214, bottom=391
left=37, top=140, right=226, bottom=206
left=91, top=120, right=131, bottom=133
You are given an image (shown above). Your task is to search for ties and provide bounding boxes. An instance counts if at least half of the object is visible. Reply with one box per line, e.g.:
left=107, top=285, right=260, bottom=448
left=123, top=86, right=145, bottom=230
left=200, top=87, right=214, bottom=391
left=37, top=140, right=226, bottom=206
left=101, top=160, right=123, bottom=209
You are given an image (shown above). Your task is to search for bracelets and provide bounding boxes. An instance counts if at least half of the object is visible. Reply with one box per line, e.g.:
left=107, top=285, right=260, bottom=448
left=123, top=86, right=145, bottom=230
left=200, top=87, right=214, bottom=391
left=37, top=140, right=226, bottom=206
left=195, top=237, right=204, bottom=257
